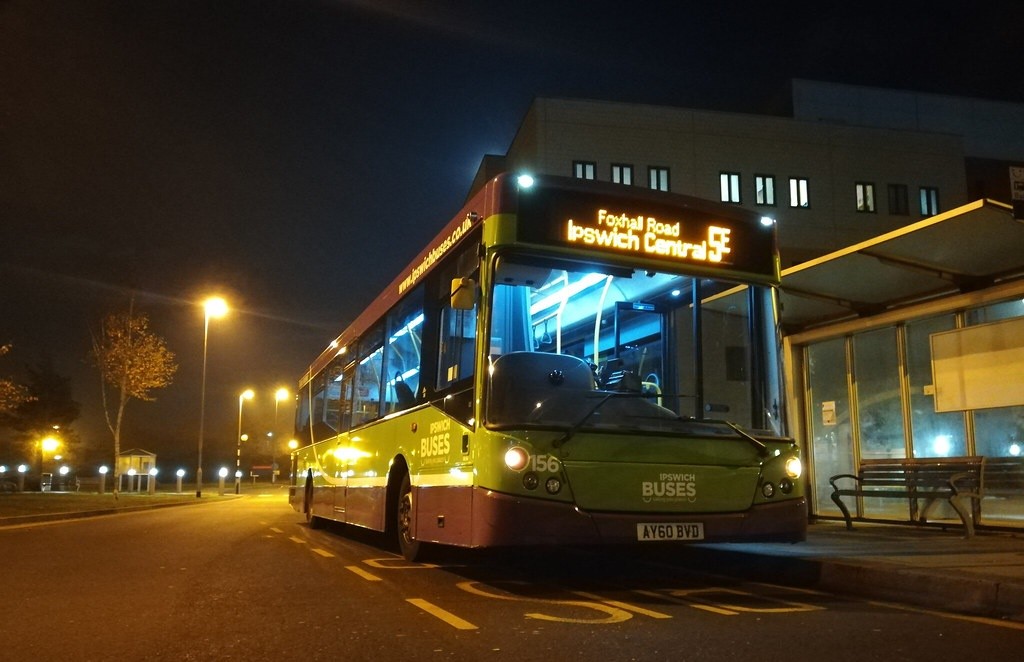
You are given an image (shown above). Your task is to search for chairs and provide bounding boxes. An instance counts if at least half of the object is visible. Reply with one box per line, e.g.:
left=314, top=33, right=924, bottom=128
left=394, top=371, right=416, bottom=407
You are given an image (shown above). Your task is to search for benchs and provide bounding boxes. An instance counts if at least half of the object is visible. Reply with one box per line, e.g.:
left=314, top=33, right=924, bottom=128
left=830, top=456, right=987, bottom=543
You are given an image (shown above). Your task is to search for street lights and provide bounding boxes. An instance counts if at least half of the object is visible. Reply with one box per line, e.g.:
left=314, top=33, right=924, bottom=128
left=272, top=388, right=298, bottom=484
left=236, top=390, right=255, bottom=495
left=195, top=297, right=230, bottom=497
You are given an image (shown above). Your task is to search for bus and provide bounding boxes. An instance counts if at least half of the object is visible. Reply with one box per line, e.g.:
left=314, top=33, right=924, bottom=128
left=289, top=169, right=809, bottom=560
